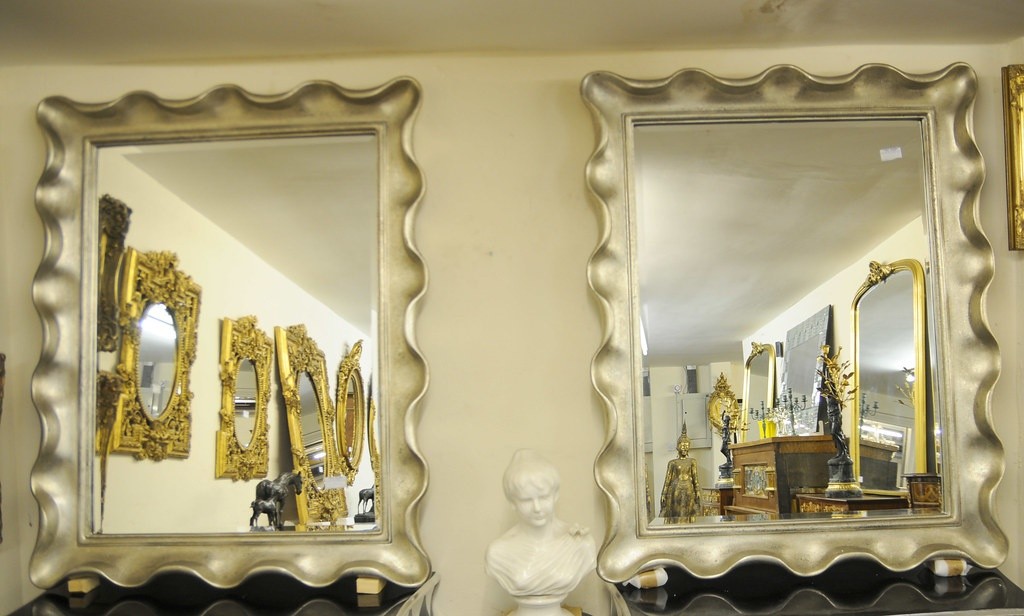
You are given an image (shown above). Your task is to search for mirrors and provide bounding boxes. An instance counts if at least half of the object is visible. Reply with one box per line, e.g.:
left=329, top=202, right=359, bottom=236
left=851, top=259, right=936, bottom=498
left=26, top=72, right=434, bottom=589
left=774, top=304, right=832, bottom=433
left=1001, top=64, right=1024, bottom=251
left=334, top=339, right=366, bottom=484
left=585, top=60, right=1011, bottom=584
left=114, top=246, right=203, bottom=462
left=215, top=314, right=274, bottom=480
left=276, top=324, right=349, bottom=526
left=740, top=341, right=776, bottom=442
left=94, top=193, right=133, bottom=455
left=367, top=397, right=376, bottom=473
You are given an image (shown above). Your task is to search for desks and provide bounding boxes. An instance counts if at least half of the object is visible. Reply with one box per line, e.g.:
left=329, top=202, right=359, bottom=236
left=701, top=487, right=733, bottom=517
left=902, top=473, right=942, bottom=509
left=727, top=435, right=847, bottom=514
left=796, top=493, right=908, bottom=512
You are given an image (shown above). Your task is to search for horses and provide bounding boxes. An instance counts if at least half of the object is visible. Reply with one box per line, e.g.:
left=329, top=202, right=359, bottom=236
left=357, top=484, right=375, bottom=514
left=249, top=471, right=303, bottom=528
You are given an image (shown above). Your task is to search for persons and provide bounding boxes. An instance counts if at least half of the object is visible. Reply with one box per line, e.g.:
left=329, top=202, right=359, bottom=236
left=483, top=448, right=598, bottom=599
left=720, top=410, right=732, bottom=466
left=823, top=381, right=848, bottom=459
left=661, top=436, right=701, bottom=517
